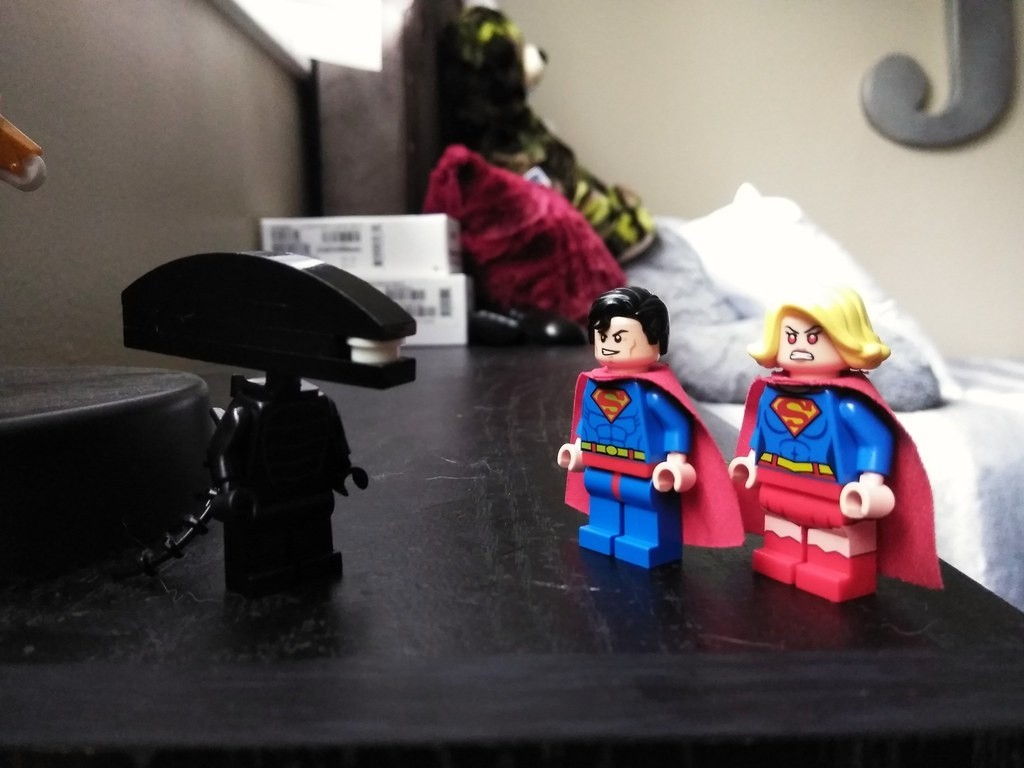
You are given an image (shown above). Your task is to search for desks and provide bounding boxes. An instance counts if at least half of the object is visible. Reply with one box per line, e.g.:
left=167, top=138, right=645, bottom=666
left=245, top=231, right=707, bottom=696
left=0, top=340, right=1024, bottom=768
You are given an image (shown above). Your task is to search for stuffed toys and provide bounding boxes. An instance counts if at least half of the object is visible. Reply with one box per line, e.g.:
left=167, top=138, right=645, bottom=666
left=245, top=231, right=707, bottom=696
left=432, top=6, right=655, bottom=266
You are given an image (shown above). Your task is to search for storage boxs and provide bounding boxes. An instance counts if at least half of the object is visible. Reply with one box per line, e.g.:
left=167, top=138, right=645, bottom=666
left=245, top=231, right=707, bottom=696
left=346, top=271, right=474, bottom=349
left=258, top=210, right=463, bottom=276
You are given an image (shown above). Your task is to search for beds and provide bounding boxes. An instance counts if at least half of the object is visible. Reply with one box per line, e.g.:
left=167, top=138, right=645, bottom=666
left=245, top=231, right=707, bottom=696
left=401, top=0, right=1024, bottom=612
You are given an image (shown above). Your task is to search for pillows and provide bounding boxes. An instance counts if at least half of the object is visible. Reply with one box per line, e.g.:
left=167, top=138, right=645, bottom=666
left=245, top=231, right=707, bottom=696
left=678, top=182, right=965, bottom=402
left=622, top=225, right=950, bottom=412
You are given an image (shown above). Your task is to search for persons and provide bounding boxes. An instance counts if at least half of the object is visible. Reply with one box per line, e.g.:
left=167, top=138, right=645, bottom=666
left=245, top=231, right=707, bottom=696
left=729, top=290, right=944, bottom=604
left=559, top=286, right=745, bottom=567
